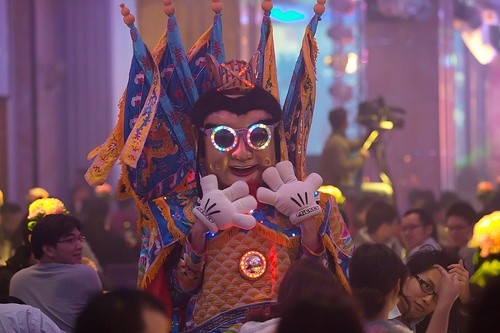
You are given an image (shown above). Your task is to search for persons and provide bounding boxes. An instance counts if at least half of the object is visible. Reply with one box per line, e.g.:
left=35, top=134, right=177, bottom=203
left=400, top=245, right=472, bottom=333
left=0, top=184, right=142, bottom=304
left=0, top=302, right=67, bottom=333
left=348, top=242, right=418, bottom=333
left=323, top=107, right=371, bottom=191
left=239, top=258, right=365, bottom=333
left=10, top=213, right=103, bottom=333
left=159, top=82, right=358, bottom=333
left=71, top=287, right=174, bottom=333
left=398, top=207, right=442, bottom=262
left=344, top=173, right=500, bottom=333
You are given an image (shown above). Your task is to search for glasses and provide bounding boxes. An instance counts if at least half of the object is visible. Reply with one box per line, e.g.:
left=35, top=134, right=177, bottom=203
left=56, top=235, right=85, bottom=244
left=443, top=224, right=470, bottom=232
left=400, top=223, right=423, bottom=232
left=414, top=274, right=439, bottom=304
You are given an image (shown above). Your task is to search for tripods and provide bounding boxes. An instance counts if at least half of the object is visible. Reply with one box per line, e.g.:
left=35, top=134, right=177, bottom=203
left=348, top=127, right=403, bottom=232
